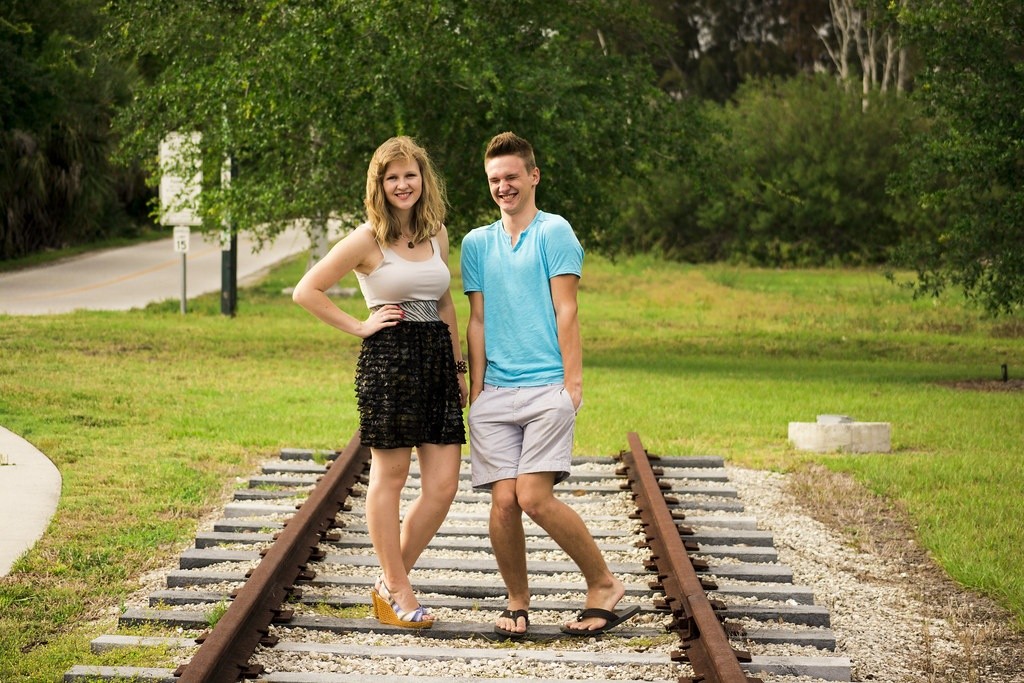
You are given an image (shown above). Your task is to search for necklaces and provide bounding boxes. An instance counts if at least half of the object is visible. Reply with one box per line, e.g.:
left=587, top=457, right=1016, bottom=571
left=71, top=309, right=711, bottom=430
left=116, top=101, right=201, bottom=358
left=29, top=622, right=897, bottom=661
left=400, top=233, right=415, bottom=249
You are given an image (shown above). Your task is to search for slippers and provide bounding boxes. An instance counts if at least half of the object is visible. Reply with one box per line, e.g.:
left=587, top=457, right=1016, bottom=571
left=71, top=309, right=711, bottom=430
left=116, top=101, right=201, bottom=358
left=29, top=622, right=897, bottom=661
left=560, top=605, right=641, bottom=634
left=494, top=609, right=528, bottom=638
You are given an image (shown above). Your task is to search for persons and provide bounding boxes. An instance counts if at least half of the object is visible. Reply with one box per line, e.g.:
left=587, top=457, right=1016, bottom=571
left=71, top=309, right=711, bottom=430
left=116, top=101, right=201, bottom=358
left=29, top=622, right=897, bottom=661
left=459, top=132, right=642, bottom=637
left=292, top=137, right=470, bottom=629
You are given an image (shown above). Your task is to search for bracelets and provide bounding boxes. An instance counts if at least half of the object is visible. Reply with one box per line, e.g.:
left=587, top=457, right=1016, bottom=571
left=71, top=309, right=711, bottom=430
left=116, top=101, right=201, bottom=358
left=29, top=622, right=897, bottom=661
left=455, top=360, right=468, bottom=374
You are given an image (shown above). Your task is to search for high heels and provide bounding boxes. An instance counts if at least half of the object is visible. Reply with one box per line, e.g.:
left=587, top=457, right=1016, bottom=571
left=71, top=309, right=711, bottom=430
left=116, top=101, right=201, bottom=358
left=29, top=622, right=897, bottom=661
left=375, top=579, right=433, bottom=628
left=371, top=576, right=382, bottom=618
